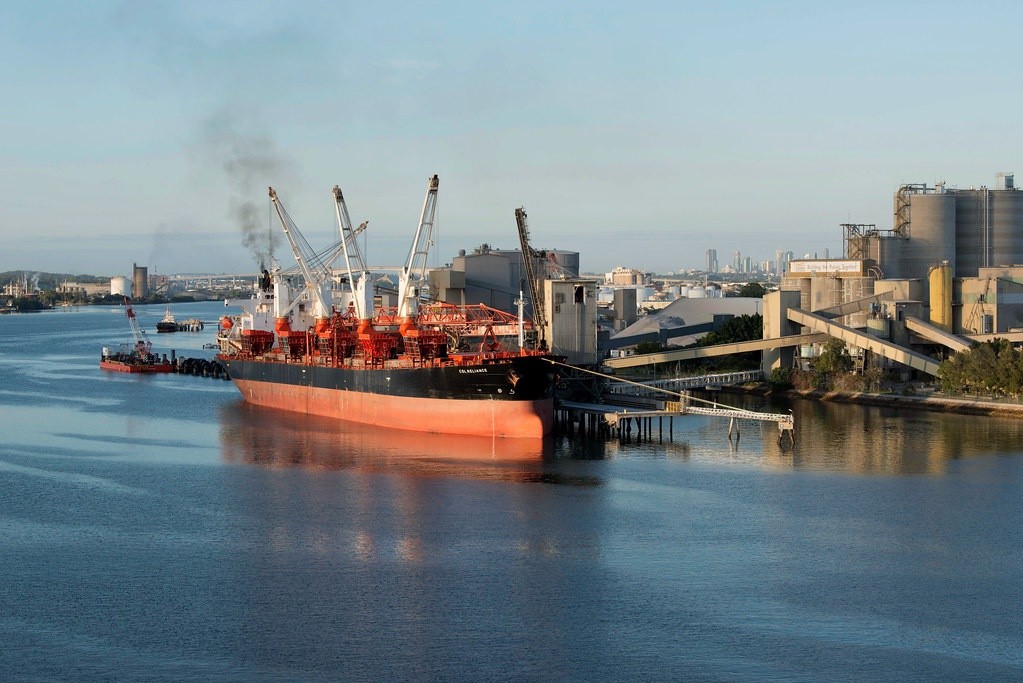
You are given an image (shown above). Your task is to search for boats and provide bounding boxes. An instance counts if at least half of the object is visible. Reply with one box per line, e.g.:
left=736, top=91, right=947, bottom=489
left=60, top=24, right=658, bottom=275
left=155, top=307, right=178, bottom=332
left=99, top=289, right=173, bottom=373
left=214, top=173, right=568, bottom=439
